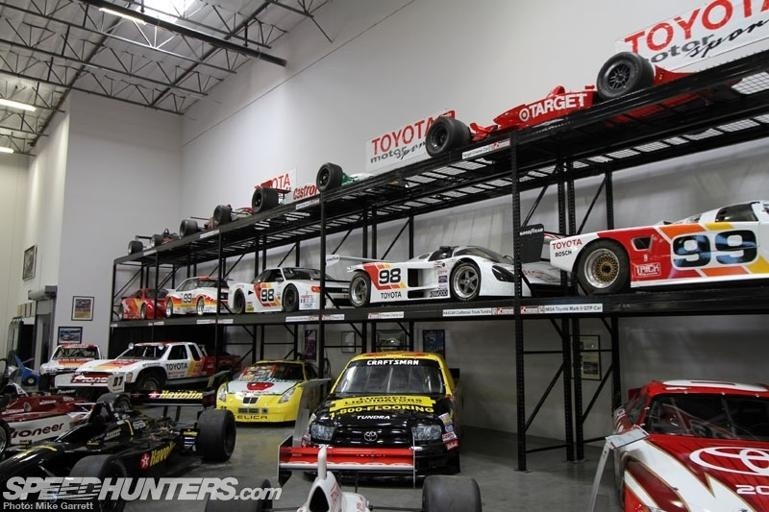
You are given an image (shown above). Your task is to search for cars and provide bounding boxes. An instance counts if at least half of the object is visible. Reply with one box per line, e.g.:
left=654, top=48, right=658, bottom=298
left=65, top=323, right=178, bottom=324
left=40, top=344, right=103, bottom=390
left=8, top=354, right=39, bottom=385
left=326, top=245, right=573, bottom=307
left=63, top=334, right=74, bottom=340
left=227, top=266, right=356, bottom=315
left=612, top=377, right=769, bottom=511
left=164, top=276, right=236, bottom=316
left=119, top=288, right=176, bottom=319
left=303, top=351, right=461, bottom=477
left=217, top=360, right=330, bottom=424
left=520, top=199, right=769, bottom=295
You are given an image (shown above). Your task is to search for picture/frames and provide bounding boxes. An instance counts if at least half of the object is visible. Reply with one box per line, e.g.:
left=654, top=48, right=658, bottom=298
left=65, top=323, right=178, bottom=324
left=304, top=329, right=318, bottom=363
left=422, top=328, right=446, bottom=360
left=21, top=244, right=37, bottom=281
left=71, top=294, right=95, bottom=322
left=563, top=334, right=603, bottom=382
left=340, top=330, right=356, bottom=353
left=371, top=328, right=408, bottom=353
left=56, top=326, right=83, bottom=345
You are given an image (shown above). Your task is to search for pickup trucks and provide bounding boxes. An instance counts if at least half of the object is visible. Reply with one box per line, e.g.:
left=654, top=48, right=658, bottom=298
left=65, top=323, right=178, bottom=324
left=73, top=341, right=241, bottom=394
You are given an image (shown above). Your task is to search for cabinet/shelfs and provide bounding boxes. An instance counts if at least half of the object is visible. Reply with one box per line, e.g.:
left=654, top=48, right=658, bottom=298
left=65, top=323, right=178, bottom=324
left=105, top=46, right=769, bottom=471
